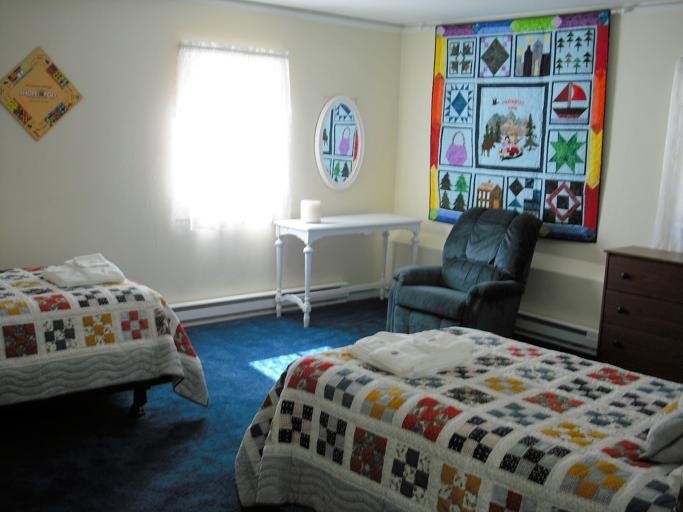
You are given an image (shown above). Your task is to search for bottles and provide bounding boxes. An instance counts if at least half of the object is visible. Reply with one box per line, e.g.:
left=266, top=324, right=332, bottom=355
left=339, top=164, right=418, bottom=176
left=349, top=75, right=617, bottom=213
left=515, top=36, right=542, bottom=77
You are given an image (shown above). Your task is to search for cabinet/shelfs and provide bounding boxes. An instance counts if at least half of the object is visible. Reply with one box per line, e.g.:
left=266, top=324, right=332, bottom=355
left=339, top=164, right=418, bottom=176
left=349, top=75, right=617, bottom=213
left=593, top=244, right=683, bottom=384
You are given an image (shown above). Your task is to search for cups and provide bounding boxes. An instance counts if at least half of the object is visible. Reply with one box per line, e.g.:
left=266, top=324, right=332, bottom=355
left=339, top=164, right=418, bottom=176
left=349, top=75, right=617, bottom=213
left=300, top=200, right=321, bottom=223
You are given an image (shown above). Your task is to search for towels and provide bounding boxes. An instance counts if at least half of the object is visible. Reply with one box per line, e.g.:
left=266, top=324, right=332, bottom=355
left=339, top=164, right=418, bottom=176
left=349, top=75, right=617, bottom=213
left=41, top=252, right=125, bottom=287
left=347, top=328, right=473, bottom=381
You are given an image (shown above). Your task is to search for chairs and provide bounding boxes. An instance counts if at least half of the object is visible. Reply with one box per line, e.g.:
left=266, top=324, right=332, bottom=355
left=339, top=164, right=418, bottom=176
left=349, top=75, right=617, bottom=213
left=385, top=207, right=543, bottom=338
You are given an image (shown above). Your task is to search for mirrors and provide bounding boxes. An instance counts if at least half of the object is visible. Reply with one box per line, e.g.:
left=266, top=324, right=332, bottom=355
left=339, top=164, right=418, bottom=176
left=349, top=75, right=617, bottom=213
left=314, top=93, right=366, bottom=193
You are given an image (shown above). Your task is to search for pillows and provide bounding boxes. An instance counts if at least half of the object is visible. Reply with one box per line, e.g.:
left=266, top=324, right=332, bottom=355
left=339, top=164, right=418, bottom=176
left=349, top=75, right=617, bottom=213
left=637, top=390, right=683, bottom=469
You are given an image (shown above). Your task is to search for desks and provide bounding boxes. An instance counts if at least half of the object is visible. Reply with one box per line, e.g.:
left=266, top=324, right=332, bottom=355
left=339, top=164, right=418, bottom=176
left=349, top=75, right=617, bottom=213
left=273, top=214, right=421, bottom=329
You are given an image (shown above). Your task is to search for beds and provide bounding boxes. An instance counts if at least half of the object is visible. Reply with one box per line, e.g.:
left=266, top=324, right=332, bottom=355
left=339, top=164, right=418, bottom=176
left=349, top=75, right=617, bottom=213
left=0, top=265, right=174, bottom=425
left=278, top=325, right=683, bottom=512
